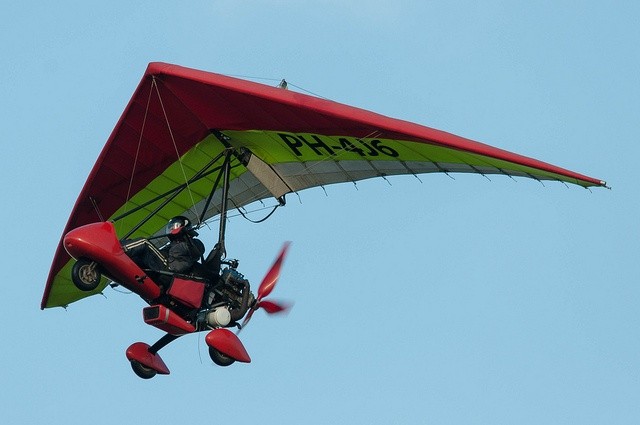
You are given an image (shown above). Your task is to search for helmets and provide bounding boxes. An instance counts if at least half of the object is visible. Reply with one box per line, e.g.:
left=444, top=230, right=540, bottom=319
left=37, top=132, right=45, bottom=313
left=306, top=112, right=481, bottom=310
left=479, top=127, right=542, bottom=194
left=168, top=216, right=191, bottom=234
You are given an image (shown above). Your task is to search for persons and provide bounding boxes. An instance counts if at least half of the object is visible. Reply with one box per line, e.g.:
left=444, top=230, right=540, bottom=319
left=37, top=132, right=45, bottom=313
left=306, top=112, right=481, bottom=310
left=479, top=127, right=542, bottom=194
left=159, top=216, right=205, bottom=274
